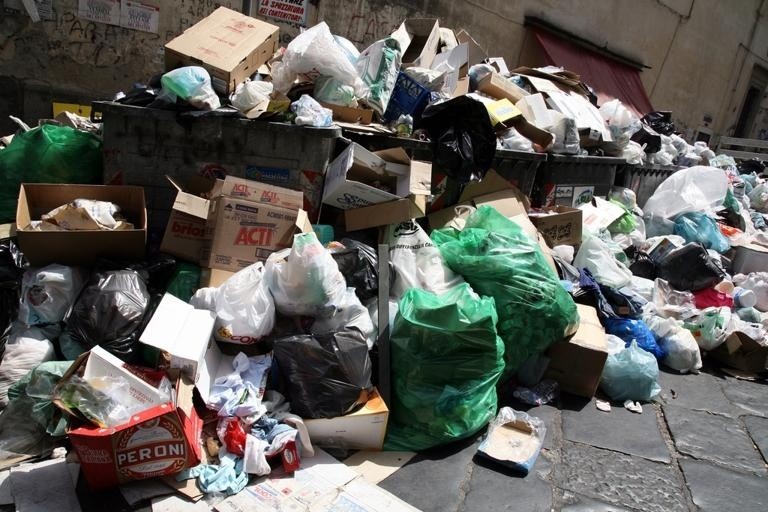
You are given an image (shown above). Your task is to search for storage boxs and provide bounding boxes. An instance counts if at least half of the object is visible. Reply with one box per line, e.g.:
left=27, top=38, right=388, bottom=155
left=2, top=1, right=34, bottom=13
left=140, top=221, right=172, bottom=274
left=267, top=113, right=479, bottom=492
left=1, top=5, right=768, bottom=498
